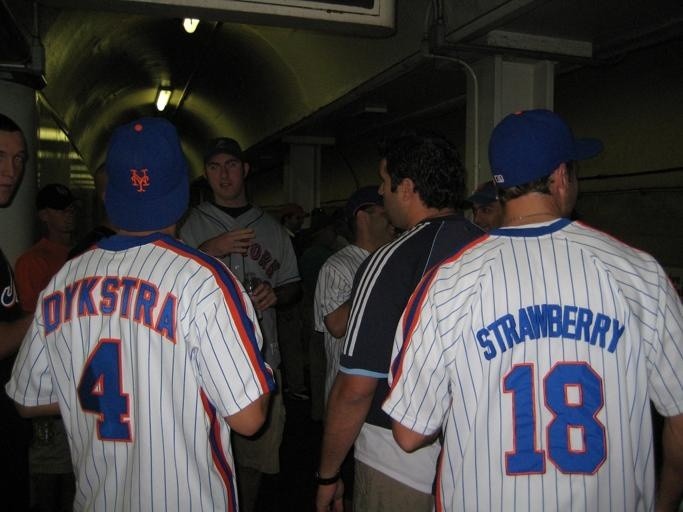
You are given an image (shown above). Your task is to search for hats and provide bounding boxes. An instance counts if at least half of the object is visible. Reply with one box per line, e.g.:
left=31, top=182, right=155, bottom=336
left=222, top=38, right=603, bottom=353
left=457, top=180, right=501, bottom=211
left=310, top=216, right=338, bottom=236
left=487, top=106, right=604, bottom=193
left=205, top=137, right=245, bottom=162
left=343, top=185, right=384, bottom=221
left=280, top=203, right=309, bottom=218
left=102, top=115, right=190, bottom=231
left=34, top=183, right=83, bottom=211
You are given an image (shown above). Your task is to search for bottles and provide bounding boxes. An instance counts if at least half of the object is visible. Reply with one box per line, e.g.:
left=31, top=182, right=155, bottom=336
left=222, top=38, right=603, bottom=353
left=243, top=272, right=261, bottom=321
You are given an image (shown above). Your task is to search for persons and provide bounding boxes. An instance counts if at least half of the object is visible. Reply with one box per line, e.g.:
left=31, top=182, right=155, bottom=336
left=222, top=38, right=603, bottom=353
left=312, top=130, right=490, bottom=512
left=64, top=161, right=108, bottom=262
left=0, top=110, right=30, bottom=510
left=11, top=182, right=92, bottom=473
left=0, top=120, right=278, bottom=512
left=379, top=106, right=681, bottom=512
left=179, top=138, right=303, bottom=512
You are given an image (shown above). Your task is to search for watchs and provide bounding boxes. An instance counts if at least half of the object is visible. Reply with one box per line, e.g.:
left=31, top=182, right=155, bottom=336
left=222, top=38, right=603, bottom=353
left=314, top=469, right=342, bottom=488
